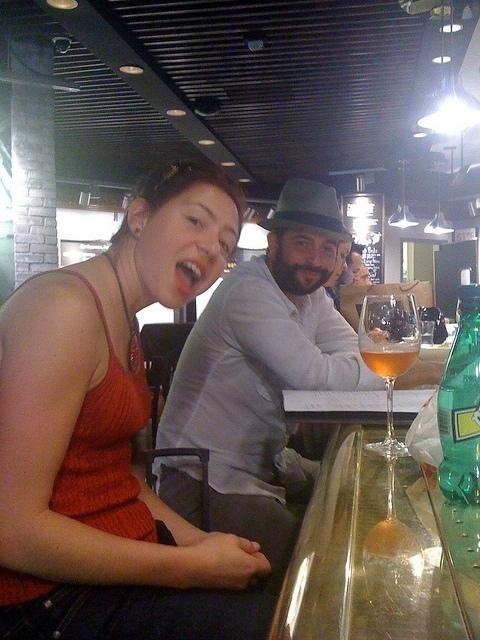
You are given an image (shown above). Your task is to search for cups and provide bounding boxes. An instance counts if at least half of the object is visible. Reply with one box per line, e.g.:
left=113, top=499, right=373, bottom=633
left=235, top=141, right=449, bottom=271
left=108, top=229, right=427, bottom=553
left=416, top=306, right=439, bottom=344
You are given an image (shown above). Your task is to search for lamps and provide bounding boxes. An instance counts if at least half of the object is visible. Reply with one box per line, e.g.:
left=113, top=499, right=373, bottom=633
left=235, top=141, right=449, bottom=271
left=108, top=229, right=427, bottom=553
left=385, top=155, right=454, bottom=234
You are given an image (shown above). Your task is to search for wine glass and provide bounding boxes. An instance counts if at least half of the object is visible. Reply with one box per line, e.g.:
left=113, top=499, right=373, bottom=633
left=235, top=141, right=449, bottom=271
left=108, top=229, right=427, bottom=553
left=359, top=294, right=422, bottom=458
left=362, top=458, right=426, bottom=616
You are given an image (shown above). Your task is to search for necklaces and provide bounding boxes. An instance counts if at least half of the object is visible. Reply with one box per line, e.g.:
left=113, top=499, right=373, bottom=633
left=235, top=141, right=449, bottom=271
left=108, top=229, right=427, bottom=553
left=101, top=249, right=144, bottom=377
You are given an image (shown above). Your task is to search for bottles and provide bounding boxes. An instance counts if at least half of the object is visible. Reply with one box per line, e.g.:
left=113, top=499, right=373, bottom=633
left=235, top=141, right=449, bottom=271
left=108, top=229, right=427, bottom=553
left=460, top=268, right=471, bottom=285
left=226, top=257, right=238, bottom=269
left=440, top=285, right=479, bottom=504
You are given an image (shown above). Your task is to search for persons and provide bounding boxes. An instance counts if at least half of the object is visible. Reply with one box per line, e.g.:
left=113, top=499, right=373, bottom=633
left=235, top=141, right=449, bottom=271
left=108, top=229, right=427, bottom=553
left=151, top=179, right=385, bottom=596
left=322, top=224, right=372, bottom=335
left=0, top=154, right=278, bottom=635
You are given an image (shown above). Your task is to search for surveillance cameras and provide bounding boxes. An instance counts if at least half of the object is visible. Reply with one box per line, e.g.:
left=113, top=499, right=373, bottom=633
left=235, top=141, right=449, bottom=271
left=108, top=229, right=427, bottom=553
left=52, top=38, right=72, bottom=53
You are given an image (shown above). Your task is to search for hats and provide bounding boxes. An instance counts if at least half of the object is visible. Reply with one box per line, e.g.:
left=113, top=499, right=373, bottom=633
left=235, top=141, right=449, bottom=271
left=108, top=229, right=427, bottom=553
left=257, top=177, right=354, bottom=241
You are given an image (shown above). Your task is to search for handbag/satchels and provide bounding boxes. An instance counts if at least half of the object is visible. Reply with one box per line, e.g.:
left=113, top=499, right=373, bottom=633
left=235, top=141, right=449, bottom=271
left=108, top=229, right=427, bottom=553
left=339, top=280, right=435, bottom=346
left=418, top=307, right=448, bottom=344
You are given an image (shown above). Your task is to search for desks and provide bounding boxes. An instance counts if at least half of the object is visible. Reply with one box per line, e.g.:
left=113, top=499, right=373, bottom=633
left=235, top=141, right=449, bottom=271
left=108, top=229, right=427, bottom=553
left=257, top=412, right=480, bottom=640
